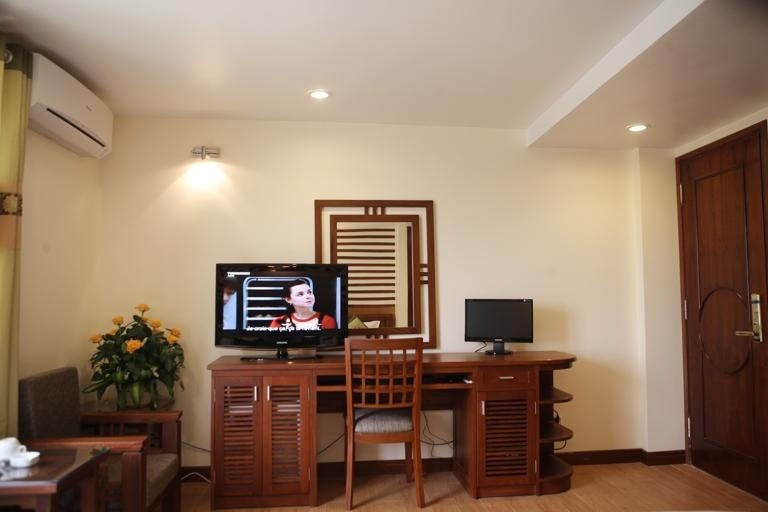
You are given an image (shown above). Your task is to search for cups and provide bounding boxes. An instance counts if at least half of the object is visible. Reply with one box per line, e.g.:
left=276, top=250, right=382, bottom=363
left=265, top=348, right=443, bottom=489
left=0, top=436, right=24, bottom=461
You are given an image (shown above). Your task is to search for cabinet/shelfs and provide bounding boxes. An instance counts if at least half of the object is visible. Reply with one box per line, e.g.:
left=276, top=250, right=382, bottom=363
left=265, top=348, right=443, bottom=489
left=477, top=356, right=576, bottom=498
left=207, top=366, right=311, bottom=510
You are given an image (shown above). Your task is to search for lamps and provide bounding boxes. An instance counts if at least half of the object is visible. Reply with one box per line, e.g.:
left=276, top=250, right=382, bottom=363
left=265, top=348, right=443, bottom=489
left=192, top=147, right=220, bottom=158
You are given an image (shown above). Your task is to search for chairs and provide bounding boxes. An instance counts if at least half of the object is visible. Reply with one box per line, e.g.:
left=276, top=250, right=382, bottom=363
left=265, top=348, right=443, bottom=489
left=344, top=338, right=426, bottom=510
left=19, top=366, right=183, bottom=511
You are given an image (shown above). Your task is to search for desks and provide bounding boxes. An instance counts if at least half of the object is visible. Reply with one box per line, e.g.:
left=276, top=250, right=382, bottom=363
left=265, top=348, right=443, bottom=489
left=0, top=447, right=111, bottom=512
left=312, top=354, right=476, bottom=505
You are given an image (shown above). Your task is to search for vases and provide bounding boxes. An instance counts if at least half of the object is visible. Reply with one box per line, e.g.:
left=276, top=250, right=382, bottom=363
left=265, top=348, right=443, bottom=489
left=126, top=383, right=152, bottom=406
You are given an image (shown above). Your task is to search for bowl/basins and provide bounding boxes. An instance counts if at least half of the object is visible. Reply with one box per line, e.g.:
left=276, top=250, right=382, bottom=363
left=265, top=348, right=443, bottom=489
left=9, top=451, right=40, bottom=467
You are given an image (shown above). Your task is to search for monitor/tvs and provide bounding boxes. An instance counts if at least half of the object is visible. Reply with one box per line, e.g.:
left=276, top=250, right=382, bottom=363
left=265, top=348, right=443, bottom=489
left=465, top=299, right=533, bottom=355
left=215, top=263, right=349, bottom=361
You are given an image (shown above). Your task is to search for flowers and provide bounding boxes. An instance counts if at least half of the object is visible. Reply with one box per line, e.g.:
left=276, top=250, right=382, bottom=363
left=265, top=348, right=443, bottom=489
left=82, top=304, right=185, bottom=412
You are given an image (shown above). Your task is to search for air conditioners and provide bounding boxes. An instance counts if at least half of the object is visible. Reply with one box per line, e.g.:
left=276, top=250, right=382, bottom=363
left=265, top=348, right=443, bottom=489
left=28, top=53, right=114, bottom=160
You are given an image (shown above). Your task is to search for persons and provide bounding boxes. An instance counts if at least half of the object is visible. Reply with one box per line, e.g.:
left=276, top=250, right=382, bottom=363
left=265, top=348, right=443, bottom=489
left=219, top=275, right=239, bottom=306
left=270, top=278, right=338, bottom=331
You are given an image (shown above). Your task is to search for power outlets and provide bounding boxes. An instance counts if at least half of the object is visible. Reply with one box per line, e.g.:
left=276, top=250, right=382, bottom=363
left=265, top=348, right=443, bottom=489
left=554, top=417, right=561, bottom=424
